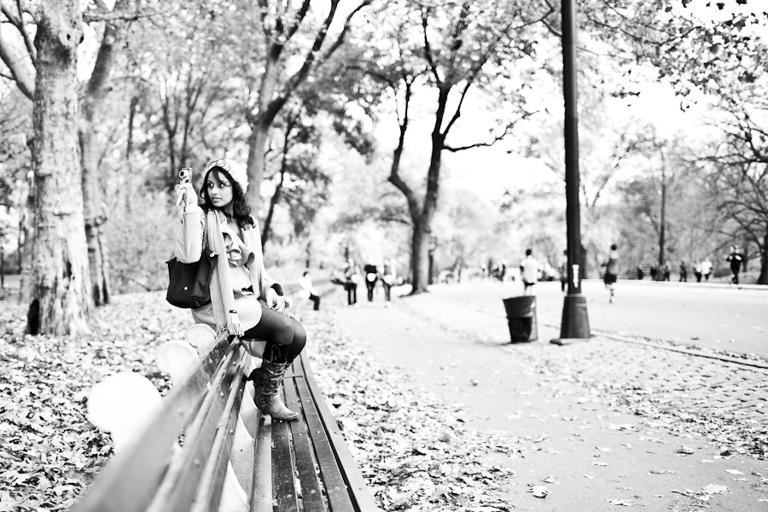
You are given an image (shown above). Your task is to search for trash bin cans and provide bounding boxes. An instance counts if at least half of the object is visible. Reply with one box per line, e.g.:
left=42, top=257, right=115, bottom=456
left=502, top=296, right=537, bottom=344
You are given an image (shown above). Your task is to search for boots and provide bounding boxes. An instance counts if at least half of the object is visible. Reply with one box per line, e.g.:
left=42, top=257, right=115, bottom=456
left=247, top=359, right=299, bottom=422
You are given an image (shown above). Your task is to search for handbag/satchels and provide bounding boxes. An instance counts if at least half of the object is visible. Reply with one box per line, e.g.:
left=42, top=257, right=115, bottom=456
left=165, top=249, right=220, bottom=309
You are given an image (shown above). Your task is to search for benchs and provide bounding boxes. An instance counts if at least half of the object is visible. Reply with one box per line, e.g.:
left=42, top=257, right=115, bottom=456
left=72, top=331, right=381, bottom=512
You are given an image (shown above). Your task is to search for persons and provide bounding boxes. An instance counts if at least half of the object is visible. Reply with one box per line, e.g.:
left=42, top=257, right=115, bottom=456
left=519, top=249, right=542, bottom=296
left=635, top=256, right=717, bottom=284
left=600, top=244, right=621, bottom=305
left=172, top=158, right=308, bottom=419
left=726, top=244, right=746, bottom=285
left=380, top=257, right=394, bottom=301
left=558, top=250, right=568, bottom=293
left=363, top=258, right=378, bottom=300
left=300, top=269, right=321, bottom=311
left=341, top=265, right=357, bottom=306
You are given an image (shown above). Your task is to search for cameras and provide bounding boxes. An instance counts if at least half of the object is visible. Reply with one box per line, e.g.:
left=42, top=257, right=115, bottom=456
left=179, top=168, right=193, bottom=185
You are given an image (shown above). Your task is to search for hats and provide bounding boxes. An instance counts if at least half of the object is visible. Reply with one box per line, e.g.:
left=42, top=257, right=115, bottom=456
left=196, top=158, right=248, bottom=198
